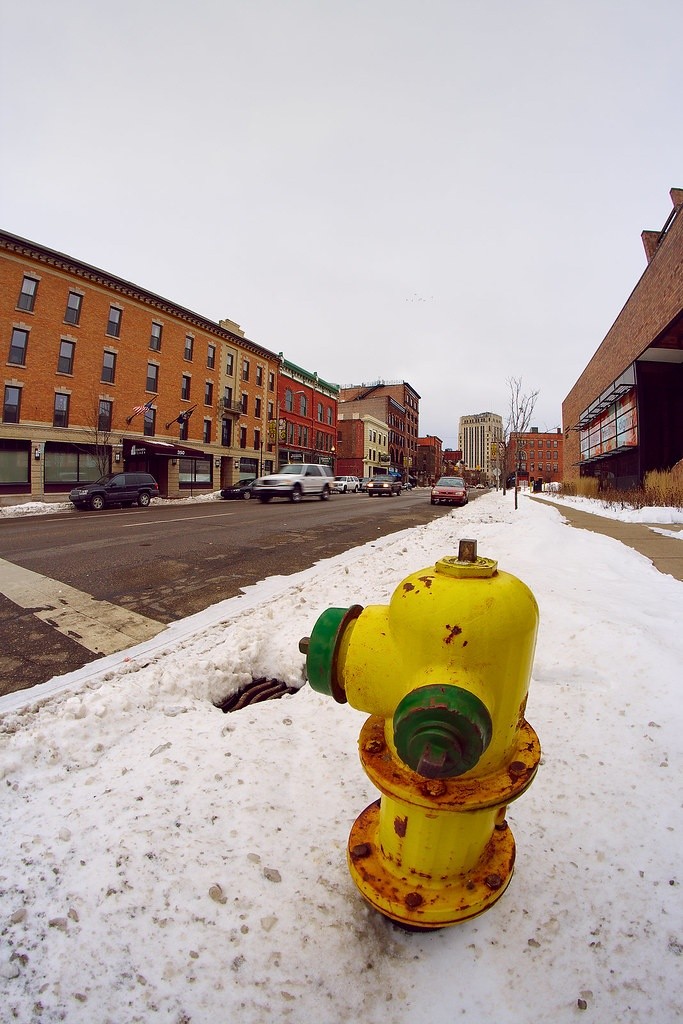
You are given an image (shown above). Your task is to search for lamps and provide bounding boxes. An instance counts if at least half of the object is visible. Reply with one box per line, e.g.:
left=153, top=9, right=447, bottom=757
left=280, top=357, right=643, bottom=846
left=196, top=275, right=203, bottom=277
left=262, top=464, right=264, bottom=469
left=35, top=448, right=40, bottom=460
left=115, top=452, right=120, bottom=463
left=216, top=460, right=219, bottom=467
left=172, top=459, right=177, bottom=465
left=235, top=462, right=238, bottom=468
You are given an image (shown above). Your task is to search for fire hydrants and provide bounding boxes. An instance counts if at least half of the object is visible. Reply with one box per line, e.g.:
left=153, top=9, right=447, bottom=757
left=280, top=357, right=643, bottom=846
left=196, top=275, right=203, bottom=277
left=297, top=537, right=544, bottom=934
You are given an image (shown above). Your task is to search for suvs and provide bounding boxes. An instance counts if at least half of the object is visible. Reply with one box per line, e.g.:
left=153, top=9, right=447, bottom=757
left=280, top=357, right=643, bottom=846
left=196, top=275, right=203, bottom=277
left=68, top=472, right=160, bottom=511
left=333, top=476, right=360, bottom=493
left=251, top=463, right=337, bottom=502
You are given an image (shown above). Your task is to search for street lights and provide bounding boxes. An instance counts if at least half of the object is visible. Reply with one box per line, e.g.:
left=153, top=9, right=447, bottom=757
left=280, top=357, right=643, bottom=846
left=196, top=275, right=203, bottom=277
left=490, top=441, right=506, bottom=491
left=276, top=390, right=305, bottom=472
left=406, top=444, right=421, bottom=482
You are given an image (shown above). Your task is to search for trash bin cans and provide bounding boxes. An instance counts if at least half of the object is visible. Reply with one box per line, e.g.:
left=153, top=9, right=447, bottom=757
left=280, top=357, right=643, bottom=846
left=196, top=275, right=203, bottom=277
left=533, top=481, right=542, bottom=492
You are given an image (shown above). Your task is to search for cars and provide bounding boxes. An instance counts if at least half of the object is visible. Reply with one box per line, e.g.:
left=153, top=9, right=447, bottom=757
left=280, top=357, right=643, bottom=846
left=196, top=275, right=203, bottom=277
left=469, top=484, right=494, bottom=489
left=220, top=478, right=258, bottom=500
left=430, top=476, right=470, bottom=506
left=402, top=482, right=412, bottom=491
left=365, top=474, right=402, bottom=497
left=359, top=477, right=370, bottom=493
